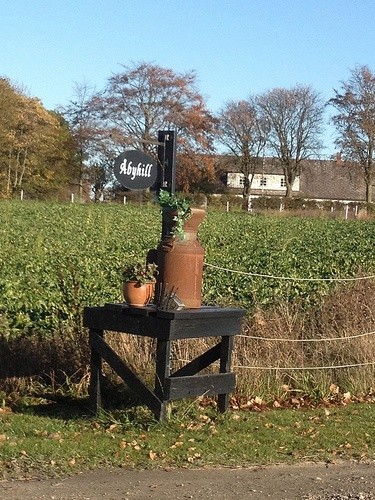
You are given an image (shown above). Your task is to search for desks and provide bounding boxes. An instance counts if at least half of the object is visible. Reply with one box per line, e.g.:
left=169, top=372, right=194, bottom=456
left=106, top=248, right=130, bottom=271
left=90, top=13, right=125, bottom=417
left=82, top=303, right=247, bottom=422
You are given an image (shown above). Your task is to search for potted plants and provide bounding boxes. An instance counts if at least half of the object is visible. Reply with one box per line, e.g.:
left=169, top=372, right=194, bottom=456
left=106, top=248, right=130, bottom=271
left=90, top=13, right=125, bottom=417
left=158, top=188, right=205, bottom=308
left=115, top=261, right=159, bottom=308
